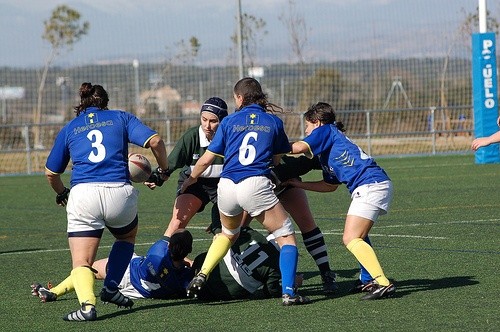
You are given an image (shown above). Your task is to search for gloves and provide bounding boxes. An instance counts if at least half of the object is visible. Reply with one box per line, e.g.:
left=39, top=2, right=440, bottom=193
left=55, top=186, right=70, bottom=207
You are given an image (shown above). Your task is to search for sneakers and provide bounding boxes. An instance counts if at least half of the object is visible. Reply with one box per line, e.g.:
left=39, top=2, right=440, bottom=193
left=63, top=303, right=97, bottom=322
left=31, top=281, right=57, bottom=302
left=362, top=282, right=388, bottom=293
left=100, top=286, right=134, bottom=307
left=322, top=272, right=337, bottom=291
left=185, top=273, right=207, bottom=298
left=361, top=284, right=396, bottom=301
left=282, top=294, right=310, bottom=306
left=347, top=279, right=365, bottom=294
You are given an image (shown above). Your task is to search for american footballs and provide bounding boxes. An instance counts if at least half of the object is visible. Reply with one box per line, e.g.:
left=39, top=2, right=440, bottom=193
left=128, top=152, right=152, bottom=183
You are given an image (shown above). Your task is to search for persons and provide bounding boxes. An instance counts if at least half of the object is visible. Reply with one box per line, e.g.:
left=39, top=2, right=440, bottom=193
left=185, top=210, right=303, bottom=302
left=271, top=152, right=340, bottom=293
left=472, top=118, right=500, bottom=150
left=179, top=77, right=312, bottom=305
left=143, top=97, right=228, bottom=241
left=45, top=82, right=169, bottom=321
left=32, top=228, right=195, bottom=303
left=282, top=103, right=396, bottom=302
